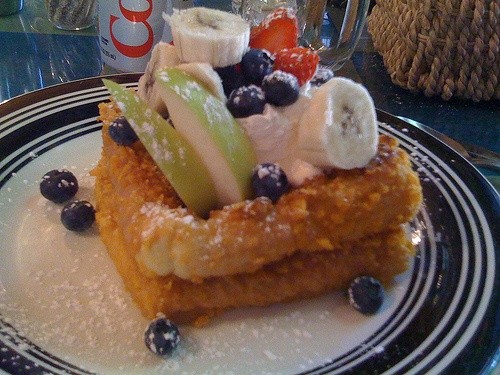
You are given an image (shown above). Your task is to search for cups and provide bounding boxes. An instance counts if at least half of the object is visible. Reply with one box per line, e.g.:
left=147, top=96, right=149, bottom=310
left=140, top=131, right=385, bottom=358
left=45, top=0, right=97, bottom=31
left=231, top=0, right=371, bottom=87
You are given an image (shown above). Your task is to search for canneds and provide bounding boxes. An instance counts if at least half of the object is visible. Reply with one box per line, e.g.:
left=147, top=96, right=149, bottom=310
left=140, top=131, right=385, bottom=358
left=99, top=0, right=174, bottom=73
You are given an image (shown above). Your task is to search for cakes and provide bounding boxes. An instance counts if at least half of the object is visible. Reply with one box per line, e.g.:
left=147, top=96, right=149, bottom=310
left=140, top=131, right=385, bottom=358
left=91, top=8, right=423, bottom=322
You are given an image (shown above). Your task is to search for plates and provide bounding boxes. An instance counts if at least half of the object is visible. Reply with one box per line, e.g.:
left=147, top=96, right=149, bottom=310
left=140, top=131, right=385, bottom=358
left=0, top=73, right=499, bottom=375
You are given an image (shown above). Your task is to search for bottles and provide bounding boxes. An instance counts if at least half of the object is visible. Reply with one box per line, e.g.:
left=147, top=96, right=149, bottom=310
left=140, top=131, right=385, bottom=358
left=97, top=0, right=167, bottom=72
